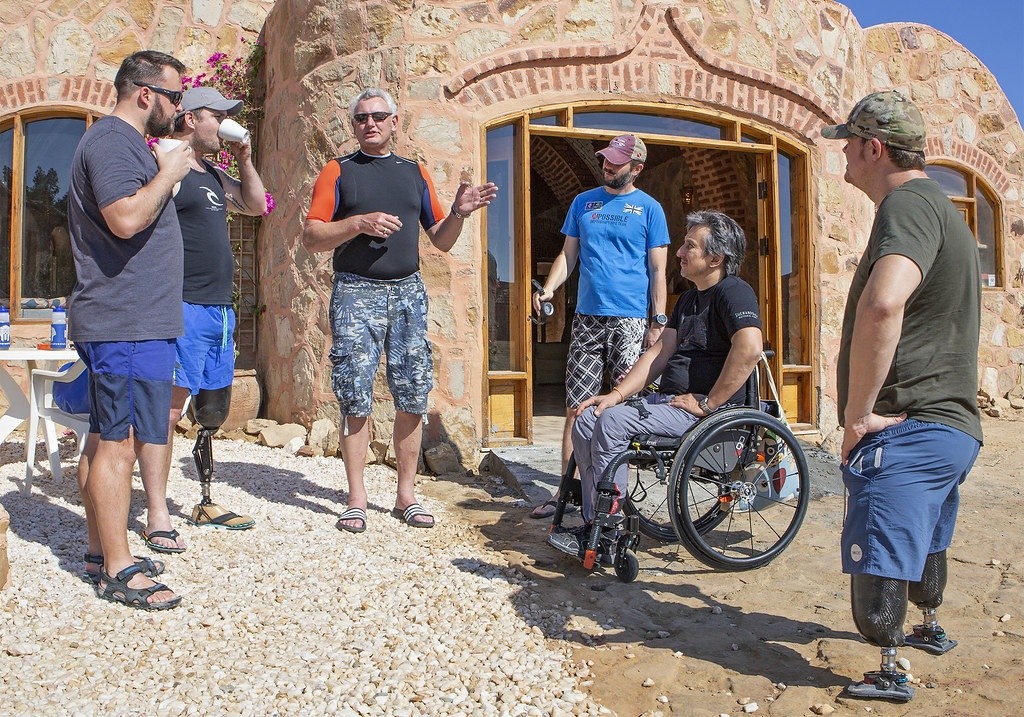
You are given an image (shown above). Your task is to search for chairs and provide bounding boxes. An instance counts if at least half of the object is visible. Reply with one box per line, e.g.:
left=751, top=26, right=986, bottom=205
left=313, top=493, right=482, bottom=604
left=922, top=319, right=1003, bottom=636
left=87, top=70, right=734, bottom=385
left=24, top=358, right=193, bottom=497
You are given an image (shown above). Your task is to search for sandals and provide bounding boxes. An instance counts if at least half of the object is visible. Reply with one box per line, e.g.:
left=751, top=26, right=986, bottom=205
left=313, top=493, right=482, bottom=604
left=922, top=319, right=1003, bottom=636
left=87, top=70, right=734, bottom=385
left=81, top=553, right=164, bottom=583
left=97, top=565, right=182, bottom=610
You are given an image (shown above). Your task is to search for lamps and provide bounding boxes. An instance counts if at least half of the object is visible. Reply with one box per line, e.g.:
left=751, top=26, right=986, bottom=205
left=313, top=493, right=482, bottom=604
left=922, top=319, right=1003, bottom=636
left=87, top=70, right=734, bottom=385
left=679, top=188, right=693, bottom=214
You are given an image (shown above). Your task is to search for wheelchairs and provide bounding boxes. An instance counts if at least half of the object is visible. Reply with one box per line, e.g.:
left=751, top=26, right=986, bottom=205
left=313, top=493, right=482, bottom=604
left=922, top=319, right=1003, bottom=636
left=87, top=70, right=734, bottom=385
left=544, top=341, right=812, bottom=584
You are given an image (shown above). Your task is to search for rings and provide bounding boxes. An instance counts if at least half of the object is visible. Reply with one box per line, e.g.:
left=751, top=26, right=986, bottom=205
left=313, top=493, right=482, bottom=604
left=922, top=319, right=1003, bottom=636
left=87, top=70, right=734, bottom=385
left=384, top=228, right=387, bottom=234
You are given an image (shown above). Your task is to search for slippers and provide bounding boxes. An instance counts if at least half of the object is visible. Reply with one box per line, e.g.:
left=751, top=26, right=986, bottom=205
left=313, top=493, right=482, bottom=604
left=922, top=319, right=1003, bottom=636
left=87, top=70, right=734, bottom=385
left=191, top=503, right=255, bottom=530
left=142, top=529, right=186, bottom=552
left=393, top=503, right=435, bottom=527
left=336, top=508, right=366, bottom=532
left=530, top=500, right=578, bottom=518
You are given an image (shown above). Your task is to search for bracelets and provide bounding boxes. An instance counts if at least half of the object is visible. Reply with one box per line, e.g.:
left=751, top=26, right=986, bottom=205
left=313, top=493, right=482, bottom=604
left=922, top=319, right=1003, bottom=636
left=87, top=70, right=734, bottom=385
left=700, top=396, right=718, bottom=414
left=451, top=204, right=471, bottom=219
left=614, top=387, right=624, bottom=402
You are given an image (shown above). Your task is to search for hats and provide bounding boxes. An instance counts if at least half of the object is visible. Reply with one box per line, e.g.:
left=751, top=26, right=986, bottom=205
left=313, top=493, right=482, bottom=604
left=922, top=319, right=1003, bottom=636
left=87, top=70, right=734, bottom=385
left=174, top=87, right=244, bottom=120
left=595, top=135, right=647, bottom=165
left=821, top=90, right=926, bottom=152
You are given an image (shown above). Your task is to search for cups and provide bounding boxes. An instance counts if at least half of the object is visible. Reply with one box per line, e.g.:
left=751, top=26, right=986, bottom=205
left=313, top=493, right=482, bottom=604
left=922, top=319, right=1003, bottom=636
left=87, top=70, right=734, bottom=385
left=157, top=138, right=192, bottom=156
left=217, top=118, right=251, bottom=144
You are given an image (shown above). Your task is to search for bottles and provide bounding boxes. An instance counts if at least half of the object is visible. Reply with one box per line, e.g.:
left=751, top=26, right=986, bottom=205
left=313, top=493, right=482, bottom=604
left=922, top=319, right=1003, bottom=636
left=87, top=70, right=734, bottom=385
left=0, top=305, right=10, bottom=350
left=50, top=305, right=67, bottom=350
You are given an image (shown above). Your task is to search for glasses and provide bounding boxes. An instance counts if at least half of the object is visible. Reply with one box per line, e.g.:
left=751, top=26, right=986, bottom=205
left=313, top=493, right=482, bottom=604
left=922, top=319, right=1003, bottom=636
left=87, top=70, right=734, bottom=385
left=132, top=80, right=183, bottom=107
left=353, top=112, right=392, bottom=123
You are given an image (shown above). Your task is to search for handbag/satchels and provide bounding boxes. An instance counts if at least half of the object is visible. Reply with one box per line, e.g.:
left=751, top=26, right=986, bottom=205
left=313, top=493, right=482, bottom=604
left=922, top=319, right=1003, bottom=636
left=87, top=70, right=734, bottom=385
left=720, top=350, right=799, bottom=513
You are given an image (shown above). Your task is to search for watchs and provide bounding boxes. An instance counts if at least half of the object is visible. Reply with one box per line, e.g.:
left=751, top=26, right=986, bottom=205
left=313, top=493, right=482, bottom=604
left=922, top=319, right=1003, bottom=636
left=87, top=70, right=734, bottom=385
left=651, top=314, right=668, bottom=326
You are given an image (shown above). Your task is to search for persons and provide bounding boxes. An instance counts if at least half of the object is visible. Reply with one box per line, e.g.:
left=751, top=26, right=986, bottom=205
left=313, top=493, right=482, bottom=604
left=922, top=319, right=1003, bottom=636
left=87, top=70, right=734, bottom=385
left=529, top=134, right=671, bottom=518
left=69, top=50, right=192, bottom=608
left=133, top=87, right=268, bottom=551
left=822, top=90, right=984, bottom=702
left=545, top=212, right=764, bottom=568
left=303, top=88, right=498, bottom=533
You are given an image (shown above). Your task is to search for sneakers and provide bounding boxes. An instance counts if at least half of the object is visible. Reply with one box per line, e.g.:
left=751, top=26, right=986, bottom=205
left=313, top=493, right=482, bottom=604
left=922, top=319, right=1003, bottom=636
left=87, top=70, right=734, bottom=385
left=552, top=525, right=615, bottom=540
left=546, top=532, right=614, bottom=563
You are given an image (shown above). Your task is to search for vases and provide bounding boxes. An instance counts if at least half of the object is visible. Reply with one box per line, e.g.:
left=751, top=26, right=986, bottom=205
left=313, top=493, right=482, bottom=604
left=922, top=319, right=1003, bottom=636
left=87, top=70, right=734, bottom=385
left=217, top=367, right=265, bottom=433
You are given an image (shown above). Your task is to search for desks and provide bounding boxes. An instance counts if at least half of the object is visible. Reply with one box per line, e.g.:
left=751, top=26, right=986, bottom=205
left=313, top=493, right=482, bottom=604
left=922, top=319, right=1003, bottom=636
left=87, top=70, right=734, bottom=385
left=1, top=349, right=82, bottom=448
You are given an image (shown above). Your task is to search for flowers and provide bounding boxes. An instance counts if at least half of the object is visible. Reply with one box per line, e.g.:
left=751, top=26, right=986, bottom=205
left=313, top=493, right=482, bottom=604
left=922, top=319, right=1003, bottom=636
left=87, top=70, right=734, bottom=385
left=142, top=36, right=275, bottom=367
